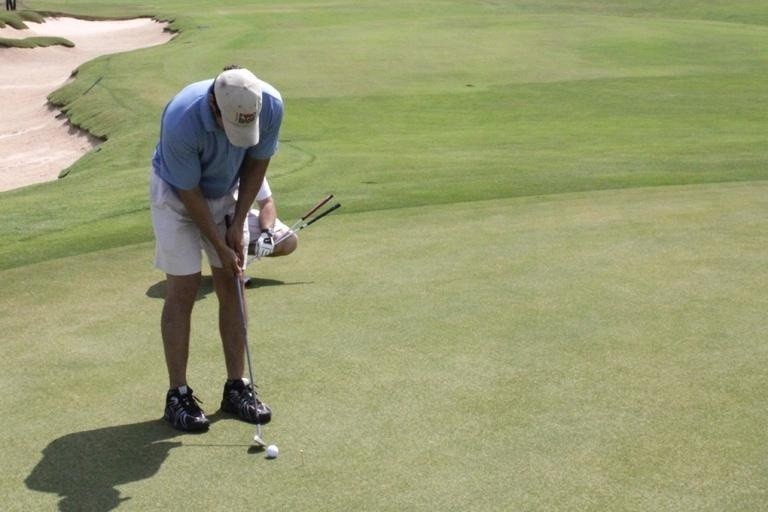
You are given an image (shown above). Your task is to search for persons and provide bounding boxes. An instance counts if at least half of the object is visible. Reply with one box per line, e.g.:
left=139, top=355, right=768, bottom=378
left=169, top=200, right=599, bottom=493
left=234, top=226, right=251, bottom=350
left=149, top=64, right=283, bottom=431
left=228, top=166, right=298, bottom=286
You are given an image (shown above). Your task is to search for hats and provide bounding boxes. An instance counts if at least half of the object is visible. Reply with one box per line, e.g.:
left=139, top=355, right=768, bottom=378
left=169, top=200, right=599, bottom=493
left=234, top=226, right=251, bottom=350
left=213, top=66, right=264, bottom=149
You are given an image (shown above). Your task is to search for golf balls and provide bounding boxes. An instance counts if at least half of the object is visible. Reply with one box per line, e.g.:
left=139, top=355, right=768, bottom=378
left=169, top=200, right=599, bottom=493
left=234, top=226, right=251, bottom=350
left=267, top=446, right=279, bottom=458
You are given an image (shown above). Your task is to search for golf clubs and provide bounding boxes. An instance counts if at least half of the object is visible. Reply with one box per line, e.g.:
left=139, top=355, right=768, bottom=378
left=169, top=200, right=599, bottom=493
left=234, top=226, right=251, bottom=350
left=224, top=214, right=267, bottom=447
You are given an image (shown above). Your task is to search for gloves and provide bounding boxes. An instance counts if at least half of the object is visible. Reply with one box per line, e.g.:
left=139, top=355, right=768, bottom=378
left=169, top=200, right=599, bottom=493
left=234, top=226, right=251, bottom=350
left=254, top=232, right=274, bottom=257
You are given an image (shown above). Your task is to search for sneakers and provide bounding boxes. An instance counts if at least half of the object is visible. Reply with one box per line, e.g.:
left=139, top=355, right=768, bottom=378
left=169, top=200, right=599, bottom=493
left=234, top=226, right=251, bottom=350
left=162, top=385, right=210, bottom=434
left=220, top=378, right=271, bottom=425
